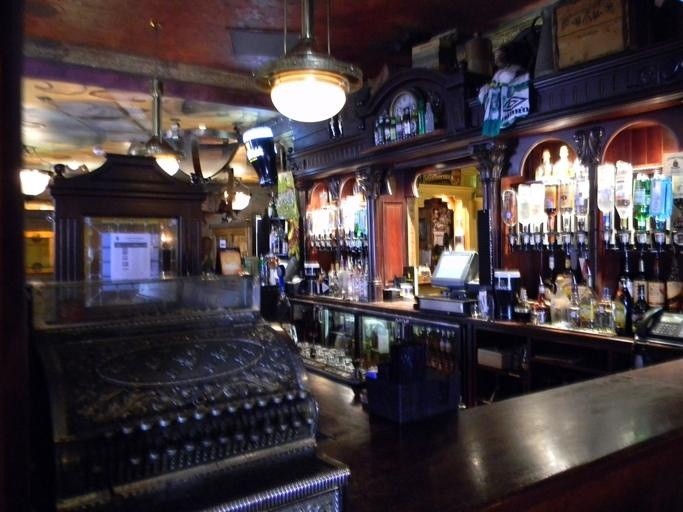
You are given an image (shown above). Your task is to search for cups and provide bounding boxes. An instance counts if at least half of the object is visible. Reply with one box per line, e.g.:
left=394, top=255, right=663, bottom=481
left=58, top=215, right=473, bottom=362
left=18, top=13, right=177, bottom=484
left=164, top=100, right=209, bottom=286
left=297, top=341, right=355, bottom=379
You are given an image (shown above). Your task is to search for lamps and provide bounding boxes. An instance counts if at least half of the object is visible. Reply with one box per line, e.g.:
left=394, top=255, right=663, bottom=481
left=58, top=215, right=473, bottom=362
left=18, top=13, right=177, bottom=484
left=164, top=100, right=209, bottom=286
left=250, top=1, right=363, bottom=126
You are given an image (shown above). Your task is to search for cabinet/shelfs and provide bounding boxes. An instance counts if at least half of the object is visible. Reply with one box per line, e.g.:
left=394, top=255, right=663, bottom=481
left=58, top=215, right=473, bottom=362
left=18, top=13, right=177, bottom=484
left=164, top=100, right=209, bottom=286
left=291, top=299, right=468, bottom=410
left=310, top=196, right=370, bottom=299
left=506, top=161, right=681, bottom=311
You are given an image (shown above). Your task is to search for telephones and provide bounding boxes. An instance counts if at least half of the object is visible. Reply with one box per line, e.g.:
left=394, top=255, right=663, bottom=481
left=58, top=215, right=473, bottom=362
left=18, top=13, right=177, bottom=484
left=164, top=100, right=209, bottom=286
left=634, top=306, right=683, bottom=340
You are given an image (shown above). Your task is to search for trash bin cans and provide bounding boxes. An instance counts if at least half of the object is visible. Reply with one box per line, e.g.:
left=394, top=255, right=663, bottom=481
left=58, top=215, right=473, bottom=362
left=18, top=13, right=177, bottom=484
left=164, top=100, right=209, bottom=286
left=260, top=285, right=284, bottom=323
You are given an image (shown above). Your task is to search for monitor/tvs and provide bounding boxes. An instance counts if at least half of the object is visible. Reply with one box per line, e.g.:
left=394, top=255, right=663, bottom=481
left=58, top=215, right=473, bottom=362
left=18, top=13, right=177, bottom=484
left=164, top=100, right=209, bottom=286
left=431, top=251, right=480, bottom=287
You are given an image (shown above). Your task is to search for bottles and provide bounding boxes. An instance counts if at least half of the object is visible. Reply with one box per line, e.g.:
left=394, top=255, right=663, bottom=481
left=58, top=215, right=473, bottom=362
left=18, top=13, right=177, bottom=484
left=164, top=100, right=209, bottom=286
left=256, top=192, right=291, bottom=287
left=411, top=324, right=453, bottom=362
left=328, top=259, right=369, bottom=303
left=500, top=161, right=683, bottom=342
left=374, top=95, right=445, bottom=147
left=310, top=224, right=370, bottom=257
